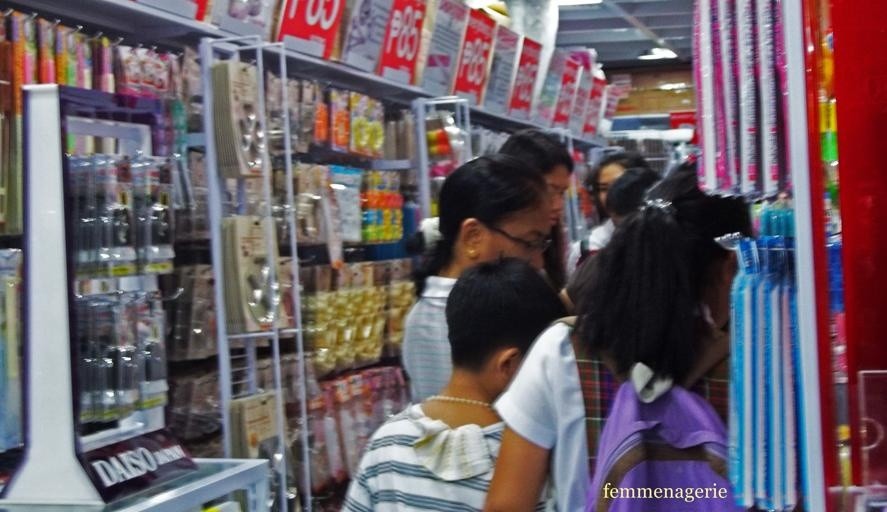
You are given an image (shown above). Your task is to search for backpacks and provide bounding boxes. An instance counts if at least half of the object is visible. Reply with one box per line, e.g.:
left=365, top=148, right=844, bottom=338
left=552, top=313, right=731, bottom=510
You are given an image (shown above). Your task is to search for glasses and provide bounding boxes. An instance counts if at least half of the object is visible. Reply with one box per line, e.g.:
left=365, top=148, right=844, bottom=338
left=484, top=224, right=552, bottom=256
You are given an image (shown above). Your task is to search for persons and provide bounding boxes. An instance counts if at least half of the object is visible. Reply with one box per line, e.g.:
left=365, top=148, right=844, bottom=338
left=343, top=254, right=570, bottom=512
left=484, top=160, right=752, bottom=512
left=497, top=129, right=573, bottom=290
left=606, top=166, right=660, bottom=228
left=589, top=150, right=649, bottom=251
left=400, top=151, right=551, bottom=403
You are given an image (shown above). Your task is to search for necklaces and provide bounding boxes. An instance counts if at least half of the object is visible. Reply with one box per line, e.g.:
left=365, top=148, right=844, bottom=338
left=428, top=395, right=492, bottom=407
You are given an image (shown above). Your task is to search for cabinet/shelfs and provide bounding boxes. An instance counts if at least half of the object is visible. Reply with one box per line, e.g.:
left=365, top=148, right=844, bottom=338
left=0, top=0, right=605, bottom=512
